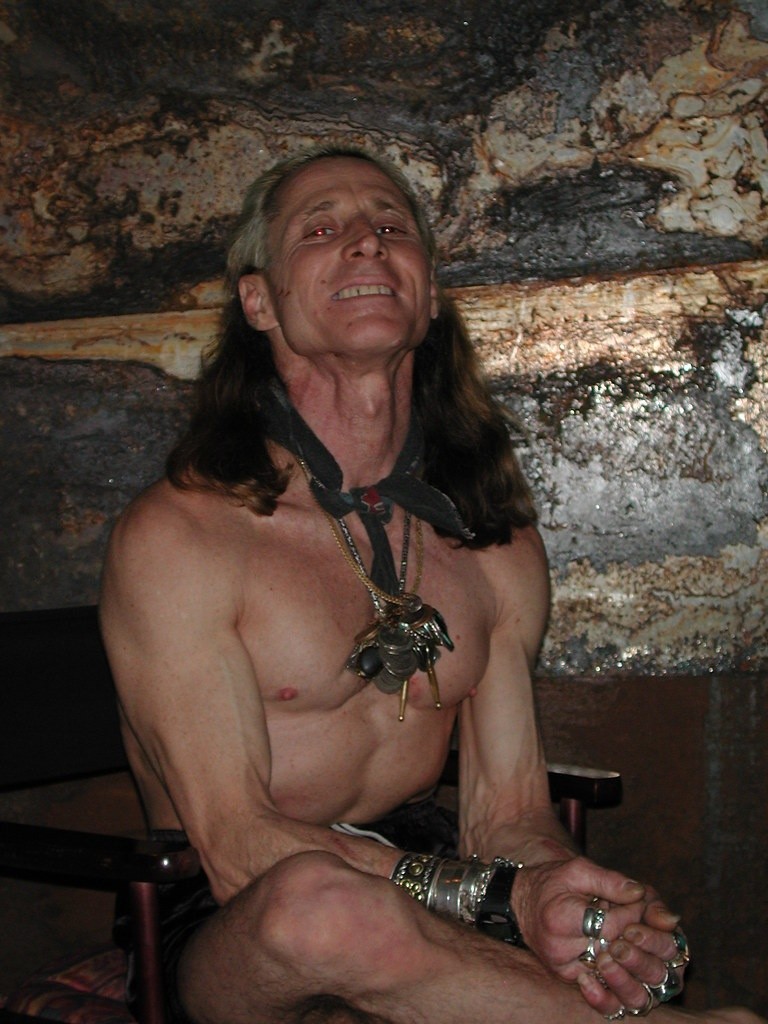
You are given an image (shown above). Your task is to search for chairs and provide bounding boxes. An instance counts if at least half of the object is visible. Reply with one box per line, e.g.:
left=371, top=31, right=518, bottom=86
left=0, top=605, right=623, bottom=1024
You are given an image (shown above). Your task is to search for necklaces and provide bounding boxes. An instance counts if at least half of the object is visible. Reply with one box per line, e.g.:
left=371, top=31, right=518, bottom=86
left=291, top=455, right=454, bottom=723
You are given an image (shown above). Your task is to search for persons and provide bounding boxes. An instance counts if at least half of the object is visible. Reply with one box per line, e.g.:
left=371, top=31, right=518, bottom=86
left=98, top=146, right=763, bottom=1024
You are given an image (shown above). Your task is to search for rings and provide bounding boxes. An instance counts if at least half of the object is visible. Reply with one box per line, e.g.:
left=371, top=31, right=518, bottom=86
left=579, top=897, right=608, bottom=989
left=603, top=929, right=689, bottom=1021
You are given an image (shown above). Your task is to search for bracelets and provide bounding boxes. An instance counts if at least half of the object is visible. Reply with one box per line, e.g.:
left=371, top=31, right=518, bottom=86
left=389, top=853, right=514, bottom=926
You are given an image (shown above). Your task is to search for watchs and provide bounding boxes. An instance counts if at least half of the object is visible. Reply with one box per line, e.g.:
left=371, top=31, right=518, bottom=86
left=475, top=859, right=523, bottom=946
